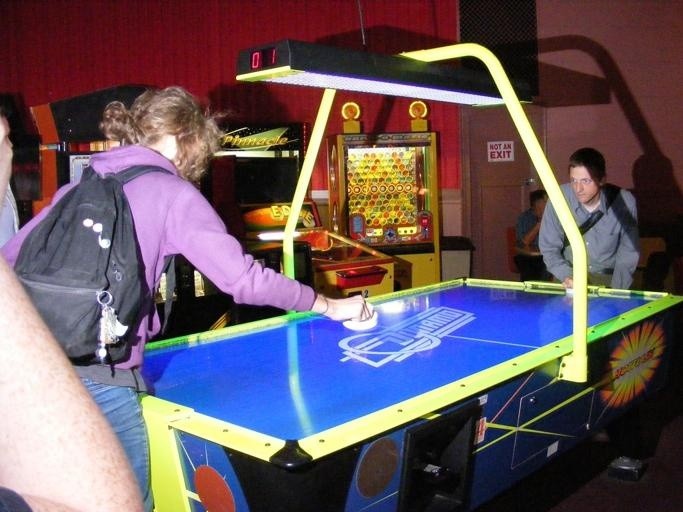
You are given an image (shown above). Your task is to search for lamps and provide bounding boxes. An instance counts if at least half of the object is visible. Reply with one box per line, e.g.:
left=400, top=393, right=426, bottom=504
left=235, top=39, right=533, bottom=108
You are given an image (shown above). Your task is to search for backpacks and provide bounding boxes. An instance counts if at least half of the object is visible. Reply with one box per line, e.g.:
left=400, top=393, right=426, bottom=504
left=13, top=164, right=171, bottom=364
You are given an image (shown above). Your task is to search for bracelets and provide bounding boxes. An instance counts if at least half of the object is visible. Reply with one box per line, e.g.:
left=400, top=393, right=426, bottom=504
left=319, top=294, right=329, bottom=315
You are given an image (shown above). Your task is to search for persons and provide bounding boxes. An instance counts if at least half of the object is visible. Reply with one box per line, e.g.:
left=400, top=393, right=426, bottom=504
left=0, top=86, right=374, bottom=512
left=511, top=190, right=546, bottom=282
left=0, top=114, right=20, bottom=251
left=0, top=255, right=145, bottom=512
left=538, top=147, right=641, bottom=290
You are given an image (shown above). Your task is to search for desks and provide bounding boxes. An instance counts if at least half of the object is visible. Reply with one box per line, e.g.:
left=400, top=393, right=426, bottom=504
left=516, top=245, right=542, bottom=257
left=134, top=277, right=683, bottom=511
